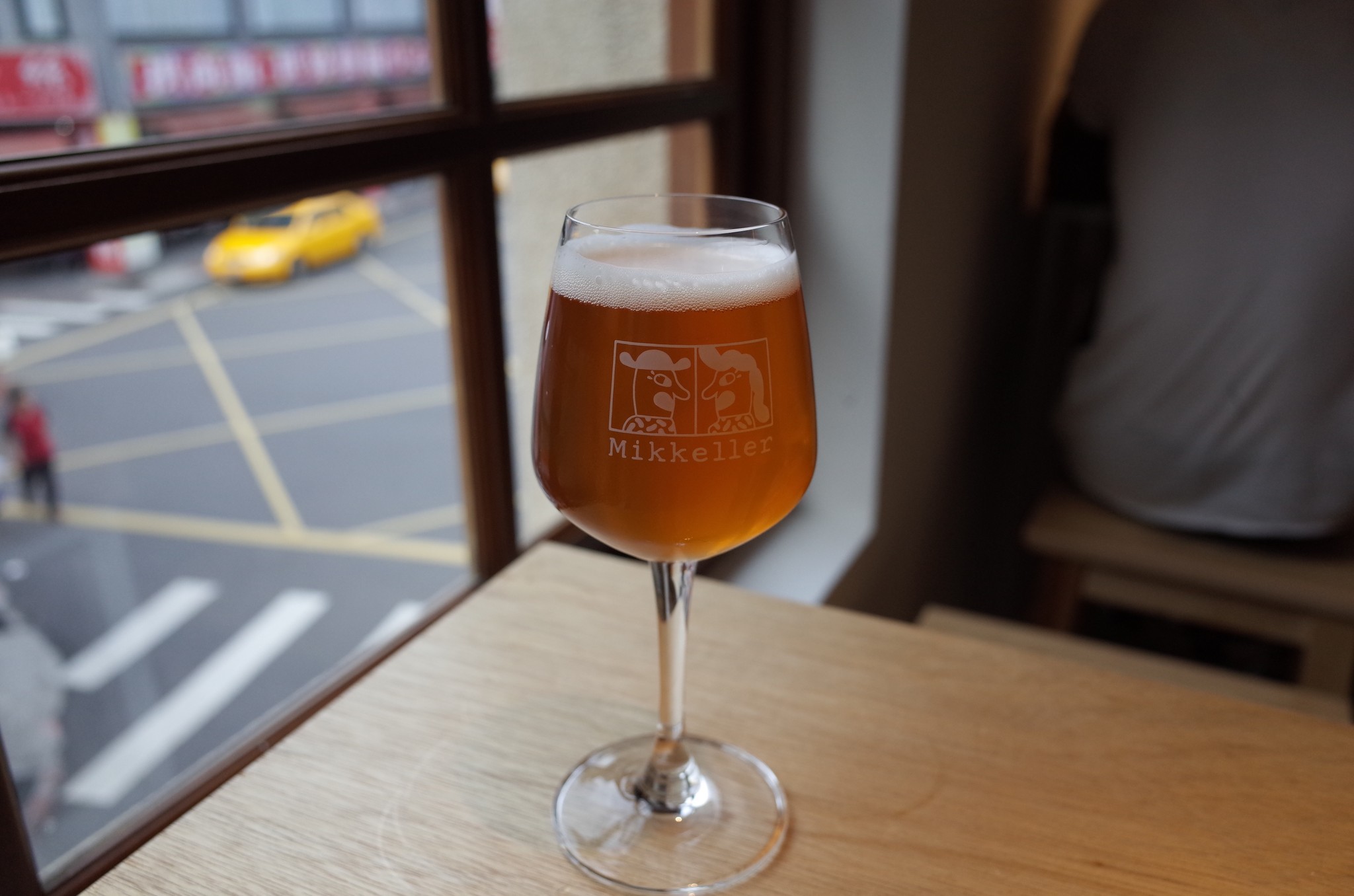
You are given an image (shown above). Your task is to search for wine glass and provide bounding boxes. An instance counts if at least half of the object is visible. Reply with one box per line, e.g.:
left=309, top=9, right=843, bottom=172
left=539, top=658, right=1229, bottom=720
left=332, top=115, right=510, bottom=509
left=530, top=194, right=818, bottom=896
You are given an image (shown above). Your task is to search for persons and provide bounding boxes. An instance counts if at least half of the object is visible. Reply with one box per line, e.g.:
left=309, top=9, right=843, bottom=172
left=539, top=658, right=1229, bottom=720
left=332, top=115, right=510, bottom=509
left=3, top=387, right=63, bottom=524
left=1032, top=0, right=1354, bottom=685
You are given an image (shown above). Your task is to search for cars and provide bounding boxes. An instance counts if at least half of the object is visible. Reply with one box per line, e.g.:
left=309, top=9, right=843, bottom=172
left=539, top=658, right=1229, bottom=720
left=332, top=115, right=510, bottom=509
left=201, top=189, right=381, bottom=287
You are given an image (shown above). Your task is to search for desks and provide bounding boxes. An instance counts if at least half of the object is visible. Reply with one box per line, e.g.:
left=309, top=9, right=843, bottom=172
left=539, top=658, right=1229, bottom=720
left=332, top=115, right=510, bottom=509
left=79, top=538, right=1354, bottom=896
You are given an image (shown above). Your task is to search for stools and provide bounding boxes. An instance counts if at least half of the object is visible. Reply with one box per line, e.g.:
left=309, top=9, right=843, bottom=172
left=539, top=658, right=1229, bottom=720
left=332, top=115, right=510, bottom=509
left=918, top=494, right=1354, bottom=725
